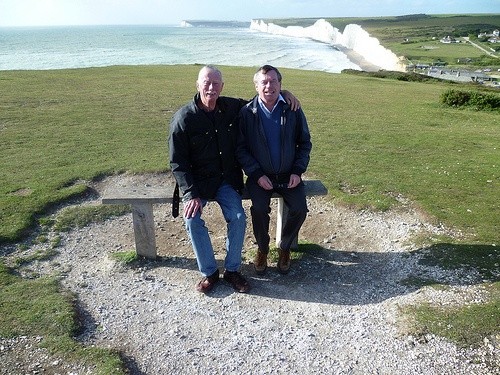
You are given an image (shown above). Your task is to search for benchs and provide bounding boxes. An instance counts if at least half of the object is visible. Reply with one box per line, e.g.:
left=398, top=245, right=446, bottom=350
left=101, top=180, right=328, bottom=259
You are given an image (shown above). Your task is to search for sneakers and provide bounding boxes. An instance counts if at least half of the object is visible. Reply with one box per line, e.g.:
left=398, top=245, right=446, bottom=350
left=223, top=271, right=250, bottom=292
left=277, top=249, right=290, bottom=275
left=196, top=269, right=219, bottom=292
left=254, top=246, right=269, bottom=273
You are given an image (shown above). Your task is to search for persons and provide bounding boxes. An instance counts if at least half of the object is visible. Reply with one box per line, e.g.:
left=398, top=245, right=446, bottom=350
left=232, top=66, right=312, bottom=277
left=168, top=63, right=301, bottom=295
